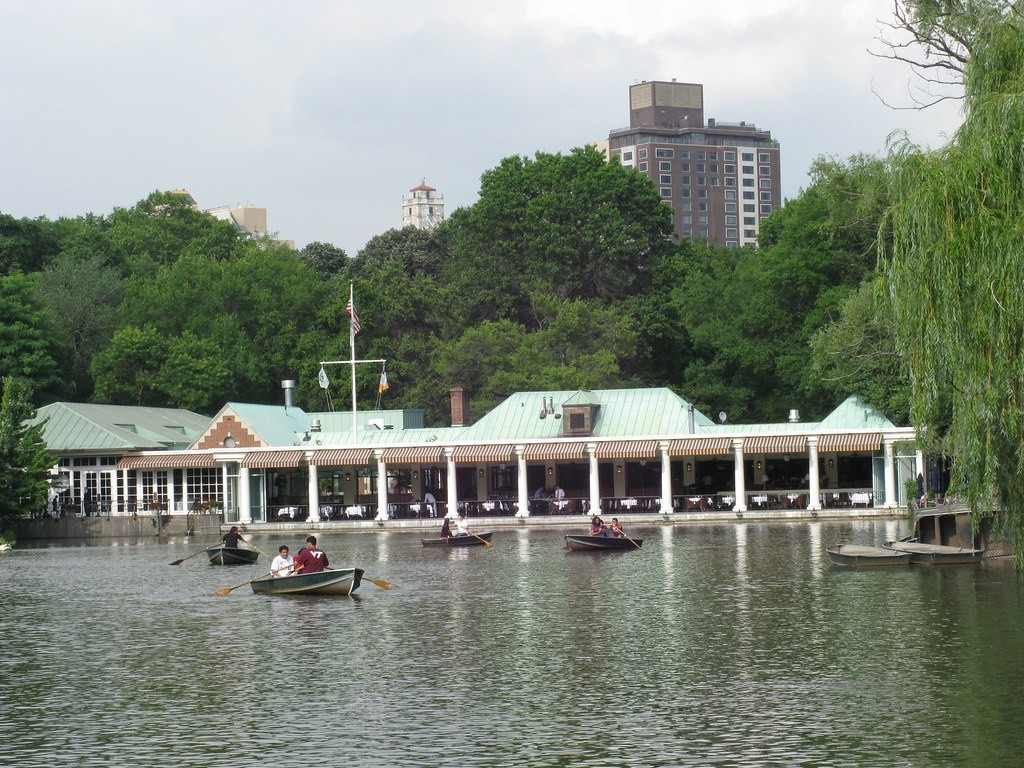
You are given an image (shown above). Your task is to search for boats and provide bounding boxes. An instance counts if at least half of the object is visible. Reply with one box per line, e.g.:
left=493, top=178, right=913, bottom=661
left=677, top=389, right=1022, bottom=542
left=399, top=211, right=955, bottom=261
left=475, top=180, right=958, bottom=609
left=250, top=567, right=364, bottom=596
left=421, top=531, right=494, bottom=547
left=884, top=541, right=982, bottom=565
left=208, top=548, right=257, bottom=565
left=825, top=543, right=911, bottom=566
left=564, top=535, right=643, bottom=551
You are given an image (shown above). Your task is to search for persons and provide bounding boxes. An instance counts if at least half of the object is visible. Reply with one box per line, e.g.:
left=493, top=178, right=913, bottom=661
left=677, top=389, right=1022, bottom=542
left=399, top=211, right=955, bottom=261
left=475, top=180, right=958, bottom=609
left=440, top=513, right=468, bottom=537
left=820, top=471, right=830, bottom=505
left=270, top=545, right=308, bottom=578
left=555, top=484, right=565, bottom=498
left=921, top=492, right=948, bottom=506
left=83, top=487, right=91, bottom=517
left=533, top=486, right=544, bottom=498
left=590, top=516, right=624, bottom=538
left=286, top=536, right=329, bottom=576
left=406, top=491, right=437, bottom=518
left=221, top=526, right=246, bottom=548
left=701, top=473, right=735, bottom=491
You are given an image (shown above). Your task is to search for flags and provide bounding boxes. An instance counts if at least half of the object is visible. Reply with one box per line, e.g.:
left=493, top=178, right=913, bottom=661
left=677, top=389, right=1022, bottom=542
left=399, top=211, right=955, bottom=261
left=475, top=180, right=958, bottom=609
left=346, top=294, right=361, bottom=339
left=319, top=367, right=329, bottom=389
left=379, top=365, right=389, bottom=393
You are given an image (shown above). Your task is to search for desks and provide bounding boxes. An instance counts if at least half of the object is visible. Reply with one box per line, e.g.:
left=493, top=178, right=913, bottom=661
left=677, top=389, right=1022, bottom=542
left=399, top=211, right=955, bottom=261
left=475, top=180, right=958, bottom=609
left=376, top=505, right=433, bottom=518
left=608, top=499, right=651, bottom=510
left=477, top=502, right=509, bottom=513
left=341, top=505, right=371, bottom=519
left=277, top=507, right=302, bottom=519
left=785, top=496, right=800, bottom=509
left=652, top=498, right=680, bottom=511
left=688, top=496, right=736, bottom=511
left=319, top=506, right=343, bottom=521
left=553, top=500, right=586, bottom=515
left=750, top=497, right=776, bottom=507
left=444, top=502, right=474, bottom=512
left=820, top=493, right=873, bottom=508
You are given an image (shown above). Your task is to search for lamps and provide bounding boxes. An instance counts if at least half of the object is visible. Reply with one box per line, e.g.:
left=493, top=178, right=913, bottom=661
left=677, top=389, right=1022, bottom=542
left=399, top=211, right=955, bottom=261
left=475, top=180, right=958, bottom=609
left=783, top=454, right=790, bottom=462
left=499, top=462, right=506, bottom=471
left=687, top=463, right=691, bottom=471
left=479, top=468, right=484, bottom=478
left=828, top=459, right=833, bottom=467
left=757, top=461, right=762, bottom=469
left=413, top=470, right=418, bottom=479
left=345, top=472, right=351, bottom=481
left=548, top=467, right=553, bottom=476
left=640, top=458, right=647, bottom=466
left=617, top=465, right=622, bottom=474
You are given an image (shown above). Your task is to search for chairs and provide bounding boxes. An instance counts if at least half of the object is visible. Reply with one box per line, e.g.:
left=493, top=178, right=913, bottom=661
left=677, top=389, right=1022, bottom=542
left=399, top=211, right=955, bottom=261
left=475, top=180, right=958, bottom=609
left=748, top=494, right=807, bottom=510
left=319, top=505, right=374, bottom=521
left=676, top=496, right=729, bottom=511
left=820, top=492, right=852, bottom=508
left=603, top=498, right=661, bottom=514
left=528, top=500, right=583, bottom=515
left=274, top=506, right=306, bottom=522
left=459, top=501, right=518, bottom=516
left=393, top=504, right=430, bottom=518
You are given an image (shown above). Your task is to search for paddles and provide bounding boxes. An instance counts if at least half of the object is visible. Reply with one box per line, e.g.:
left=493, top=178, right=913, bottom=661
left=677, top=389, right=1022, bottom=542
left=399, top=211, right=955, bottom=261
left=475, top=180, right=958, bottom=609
left=325, top=567, right=399, bottom=589
left=461, top=526, right=492, bottom=545
left=214, top=563, right=297, bottom=595
left=244, top=541, right=274, bottom=561
left=612, top=524, right=642, bottom=549
left=169, top=541, right=226, bottom=565
